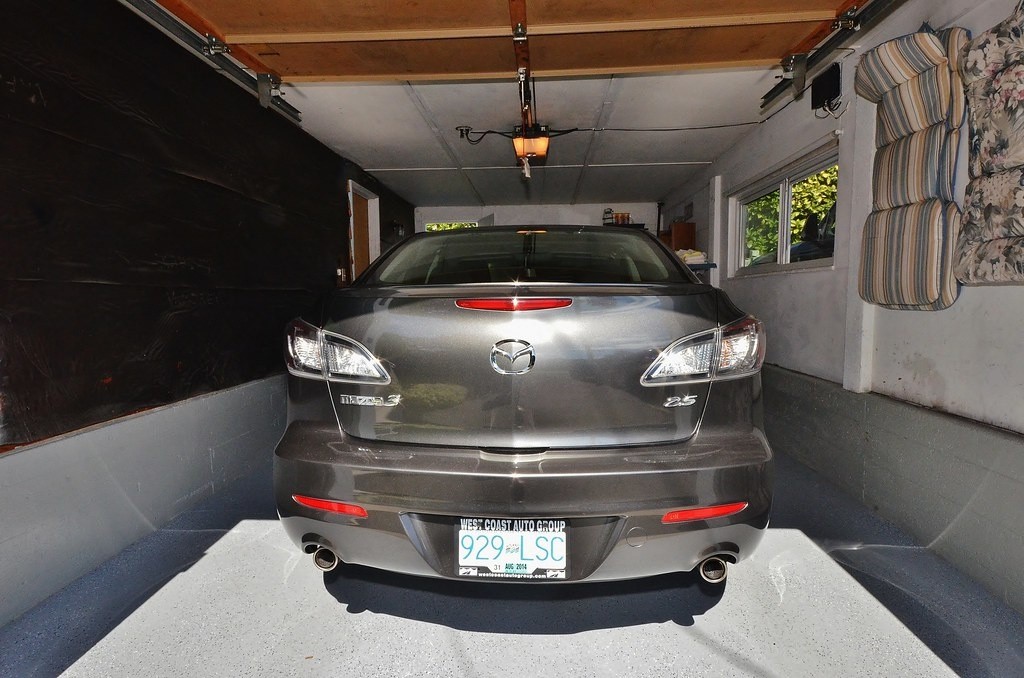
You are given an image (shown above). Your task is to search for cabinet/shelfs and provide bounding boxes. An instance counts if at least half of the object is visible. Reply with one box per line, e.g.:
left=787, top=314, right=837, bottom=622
left=657, top=222, right=695, bottom=252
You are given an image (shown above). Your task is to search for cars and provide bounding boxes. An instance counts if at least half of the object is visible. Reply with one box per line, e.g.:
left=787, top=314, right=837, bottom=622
left=274, top=225, right=775, bottom=584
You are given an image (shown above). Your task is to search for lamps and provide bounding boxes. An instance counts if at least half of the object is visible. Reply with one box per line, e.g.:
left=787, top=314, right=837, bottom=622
left=512, top=123, right=552, bottom=167
